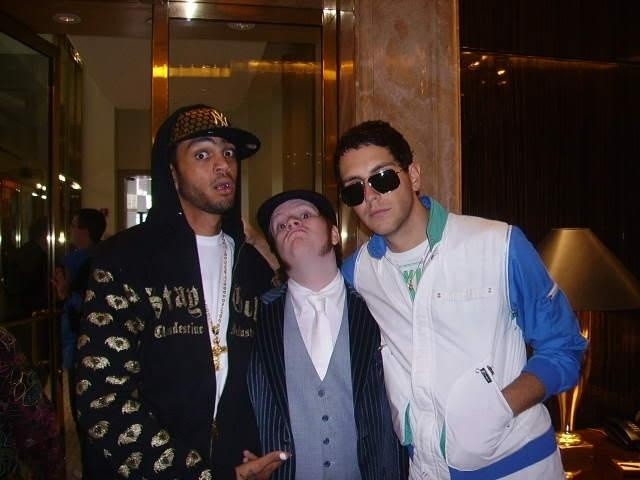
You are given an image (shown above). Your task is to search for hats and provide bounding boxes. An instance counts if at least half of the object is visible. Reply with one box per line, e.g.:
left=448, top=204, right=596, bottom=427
left=152, top=105, right=259, bottom=164
left=256, top=190, right=337, bottom=231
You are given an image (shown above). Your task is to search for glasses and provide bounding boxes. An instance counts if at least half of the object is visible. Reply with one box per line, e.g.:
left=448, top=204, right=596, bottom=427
left=339, top=168, right=403, bottom=208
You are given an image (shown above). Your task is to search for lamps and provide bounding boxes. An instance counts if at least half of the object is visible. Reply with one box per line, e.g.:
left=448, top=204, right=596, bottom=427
left=536, top=225, right=637, bottom=447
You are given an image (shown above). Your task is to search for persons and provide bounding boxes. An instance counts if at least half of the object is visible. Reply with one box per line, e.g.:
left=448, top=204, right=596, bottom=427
left=334, top=117, right=588, bottom=480
left=72, top=103, right=313, bottom=480
left=53, top=207, right=107, bottom=480
left=245, top=185, right=407, bottom=480
left=0, top=325, right=67, bottom=480
left=5, top=216, right=52, bottom=389
